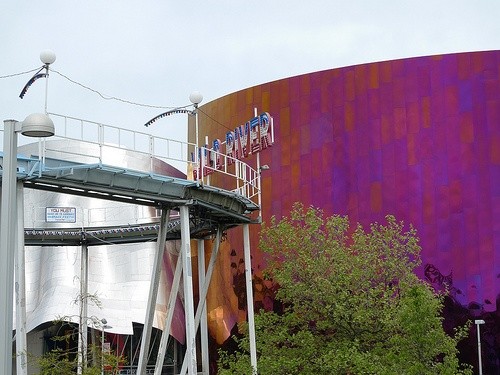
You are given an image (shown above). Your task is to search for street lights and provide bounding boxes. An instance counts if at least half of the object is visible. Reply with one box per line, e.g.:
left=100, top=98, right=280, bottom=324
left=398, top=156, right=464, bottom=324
left=189, top=93, right=203, bottom=187
left=474, top=320, right=486, bottom=375
left=39, top=49, right=56, bottom=172
